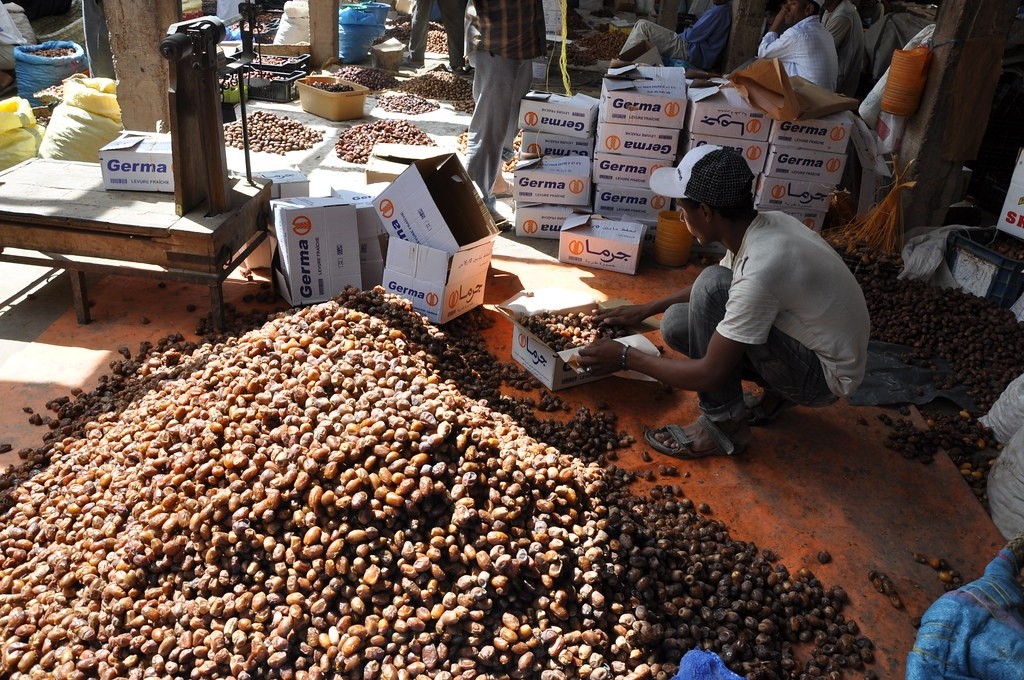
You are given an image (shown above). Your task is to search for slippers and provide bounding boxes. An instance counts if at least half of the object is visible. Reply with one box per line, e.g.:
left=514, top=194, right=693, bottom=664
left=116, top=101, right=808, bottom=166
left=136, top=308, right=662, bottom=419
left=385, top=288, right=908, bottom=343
left=399, top=55, right=425, bottom=69
left=447, top=66, right=465, bottom=74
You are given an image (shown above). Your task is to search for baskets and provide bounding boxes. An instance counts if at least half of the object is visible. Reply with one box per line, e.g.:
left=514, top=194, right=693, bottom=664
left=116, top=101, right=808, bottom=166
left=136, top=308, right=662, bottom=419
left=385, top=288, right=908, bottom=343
left=229, top=65, right=306, bottom=103
left=226, top=50, right=310, bottom=74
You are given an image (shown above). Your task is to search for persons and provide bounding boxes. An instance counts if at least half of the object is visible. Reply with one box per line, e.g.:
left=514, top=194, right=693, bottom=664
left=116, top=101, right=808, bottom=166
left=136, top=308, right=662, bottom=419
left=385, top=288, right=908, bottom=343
left=403, top=0, right=467, bottom=74
left=814, top=0, right=863, bottom=96
left=617, top=0, right=732, bottom=71
left=756, top=0, right=837, bottom=93
left=462, top=0, right=547, bottom=232
left=576, top=146, right=871, bottom=460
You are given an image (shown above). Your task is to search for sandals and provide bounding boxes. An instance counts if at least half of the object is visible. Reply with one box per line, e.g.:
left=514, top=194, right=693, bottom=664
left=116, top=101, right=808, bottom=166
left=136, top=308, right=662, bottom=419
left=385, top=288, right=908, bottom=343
left=746, top=389, right=799, bottom=424
left=644, top=415, right=747, bottom=456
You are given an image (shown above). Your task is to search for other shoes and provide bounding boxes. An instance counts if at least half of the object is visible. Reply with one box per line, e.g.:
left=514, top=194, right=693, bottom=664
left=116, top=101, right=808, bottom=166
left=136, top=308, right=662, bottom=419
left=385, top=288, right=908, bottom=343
left=492, top=182, right=514, bottom=198
left=492, top=215, right=512, bottom=230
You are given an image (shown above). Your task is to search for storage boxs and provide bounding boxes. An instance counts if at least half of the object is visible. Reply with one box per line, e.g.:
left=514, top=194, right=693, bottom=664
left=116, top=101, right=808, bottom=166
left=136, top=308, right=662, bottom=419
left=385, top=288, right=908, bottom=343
left=98, top=130, right=176, bottom=193
left=514, top=58, right=892, bottom=276
left=227, top=41, right=370, bottom=121
left=943, top=139, right=1024, bottom=324
left=241, top=141, right=502, bottom=323
left=495, top=282, right=662, bottom=391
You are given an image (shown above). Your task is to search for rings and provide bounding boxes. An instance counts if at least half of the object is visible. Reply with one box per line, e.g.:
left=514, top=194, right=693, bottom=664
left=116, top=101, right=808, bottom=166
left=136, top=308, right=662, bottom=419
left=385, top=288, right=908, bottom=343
left=585, top=365, right=594, bottom=374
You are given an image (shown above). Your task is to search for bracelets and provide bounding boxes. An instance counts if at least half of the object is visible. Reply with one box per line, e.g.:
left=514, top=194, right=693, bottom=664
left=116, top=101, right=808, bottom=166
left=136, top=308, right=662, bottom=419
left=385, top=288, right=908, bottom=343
left=621, top=344, right=632, bottom=372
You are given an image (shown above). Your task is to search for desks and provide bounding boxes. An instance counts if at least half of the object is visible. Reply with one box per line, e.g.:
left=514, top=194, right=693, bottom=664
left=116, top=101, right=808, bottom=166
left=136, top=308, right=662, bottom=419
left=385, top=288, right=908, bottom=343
left=0, top=158, right=273, bottom=337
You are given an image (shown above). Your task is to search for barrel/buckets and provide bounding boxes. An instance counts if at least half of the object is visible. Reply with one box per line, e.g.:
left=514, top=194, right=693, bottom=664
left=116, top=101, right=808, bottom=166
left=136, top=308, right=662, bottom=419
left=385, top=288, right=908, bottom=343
left=370, top=46, right=404, bottom=72
left=881, top=47, right=932, bottom=117
left=653, top=211, right=694, bottom=268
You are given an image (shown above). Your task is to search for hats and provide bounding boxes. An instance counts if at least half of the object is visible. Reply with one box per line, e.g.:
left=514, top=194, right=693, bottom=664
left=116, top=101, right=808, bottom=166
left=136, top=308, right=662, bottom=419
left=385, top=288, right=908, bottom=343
left=650, top=144, right=753, bottom=207
